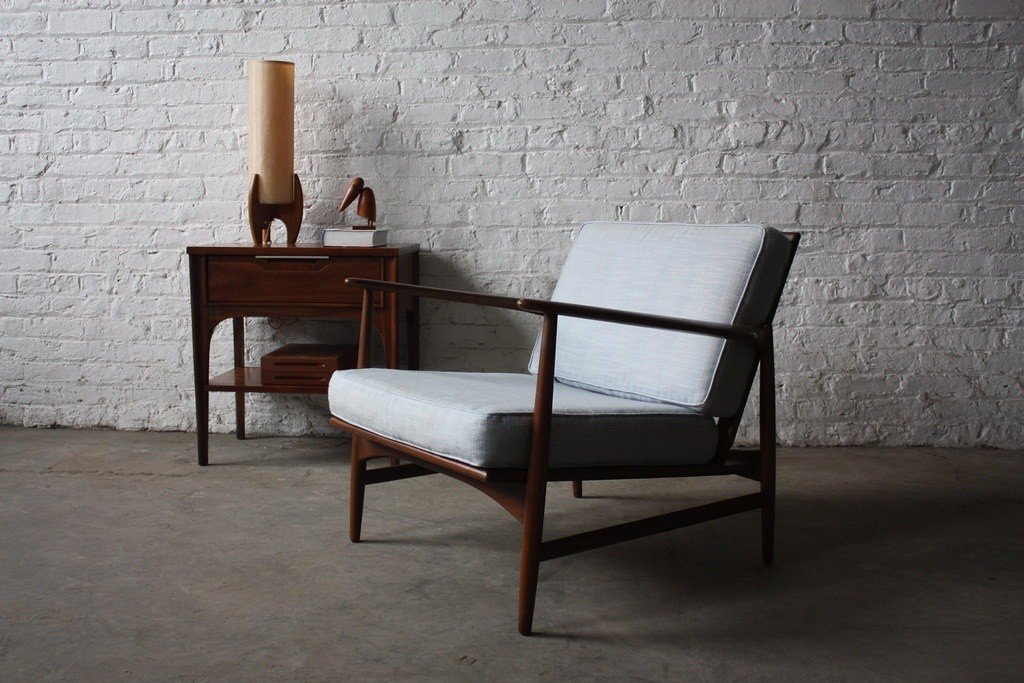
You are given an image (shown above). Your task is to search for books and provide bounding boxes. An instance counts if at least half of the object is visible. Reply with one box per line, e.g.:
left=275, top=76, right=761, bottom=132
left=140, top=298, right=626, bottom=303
left=322, top=226, right=389, bottom=247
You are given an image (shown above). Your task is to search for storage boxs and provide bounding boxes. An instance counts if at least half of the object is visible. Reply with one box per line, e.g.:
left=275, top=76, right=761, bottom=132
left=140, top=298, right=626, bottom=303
left=260, top=343, right=360, bottom=385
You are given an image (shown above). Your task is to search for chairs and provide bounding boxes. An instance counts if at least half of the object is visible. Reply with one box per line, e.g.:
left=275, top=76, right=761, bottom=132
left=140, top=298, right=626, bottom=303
left=328, top=220, right=803, bottom=637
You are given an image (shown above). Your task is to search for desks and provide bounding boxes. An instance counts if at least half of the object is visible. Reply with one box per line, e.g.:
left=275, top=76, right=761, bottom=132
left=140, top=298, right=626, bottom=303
left=186, top=243, right=422, bottom=467
